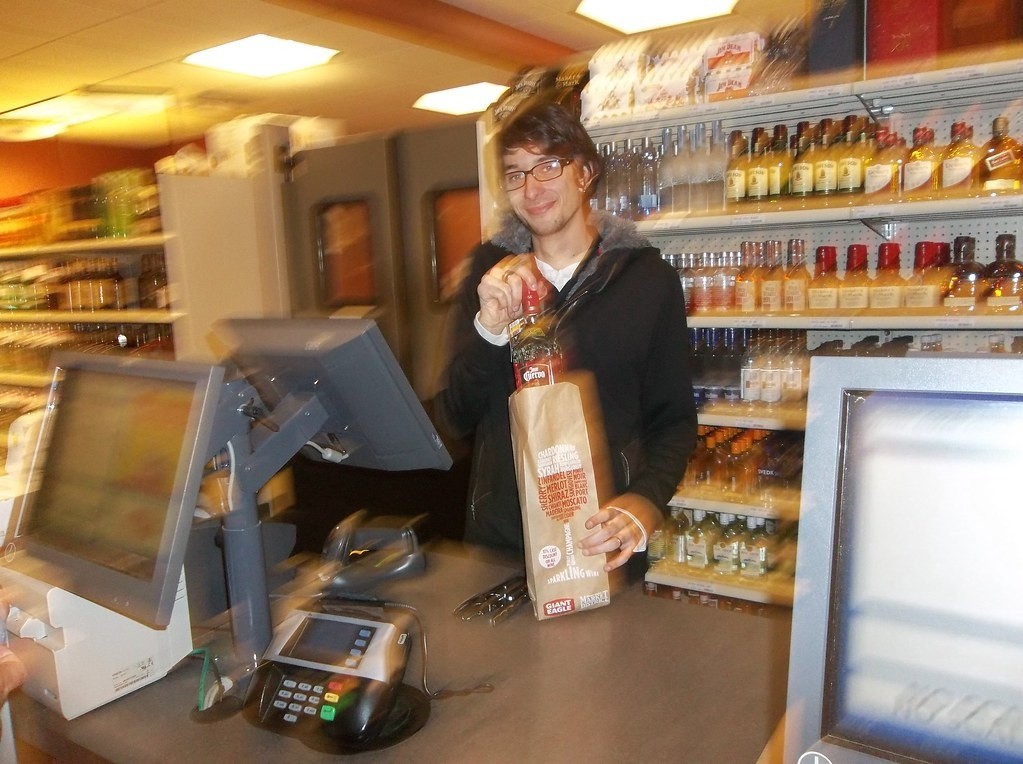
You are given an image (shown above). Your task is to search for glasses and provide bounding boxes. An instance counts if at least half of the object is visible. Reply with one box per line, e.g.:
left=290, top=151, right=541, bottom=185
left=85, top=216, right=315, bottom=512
left=500, top=159, right=573, bottom=192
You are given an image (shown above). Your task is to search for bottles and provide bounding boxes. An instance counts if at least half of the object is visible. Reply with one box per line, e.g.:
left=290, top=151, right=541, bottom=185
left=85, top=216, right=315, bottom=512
left=0, top=167, right=174, bottom=348
left=581, top=44, right=1023, bottom=581
left=512, top=277, right=565, bottom=393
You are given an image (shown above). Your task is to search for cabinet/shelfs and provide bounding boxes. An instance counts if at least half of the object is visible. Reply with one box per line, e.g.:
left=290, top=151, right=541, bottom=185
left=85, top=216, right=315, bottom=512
left=0, top=41, right=1023, bottom=620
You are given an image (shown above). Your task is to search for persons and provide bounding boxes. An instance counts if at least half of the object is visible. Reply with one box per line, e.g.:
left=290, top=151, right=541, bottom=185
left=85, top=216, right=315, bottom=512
left=439, top=103, right=697, bottom=590
left=0, top=645, right=30, bottom=709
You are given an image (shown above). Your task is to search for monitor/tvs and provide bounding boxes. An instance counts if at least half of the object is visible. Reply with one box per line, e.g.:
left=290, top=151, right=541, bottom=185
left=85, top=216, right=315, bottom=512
left=783, top=357, right=1022, bottom=763
left=216, top=307, right=455, bottom=471
left=1, top=347, right=227, bottom=629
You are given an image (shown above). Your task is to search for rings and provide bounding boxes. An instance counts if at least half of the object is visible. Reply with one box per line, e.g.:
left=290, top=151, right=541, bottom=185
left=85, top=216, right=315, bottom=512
left=613, top=535, right=622, bottom=548
left=501, top=270, right=515, bottom=283
